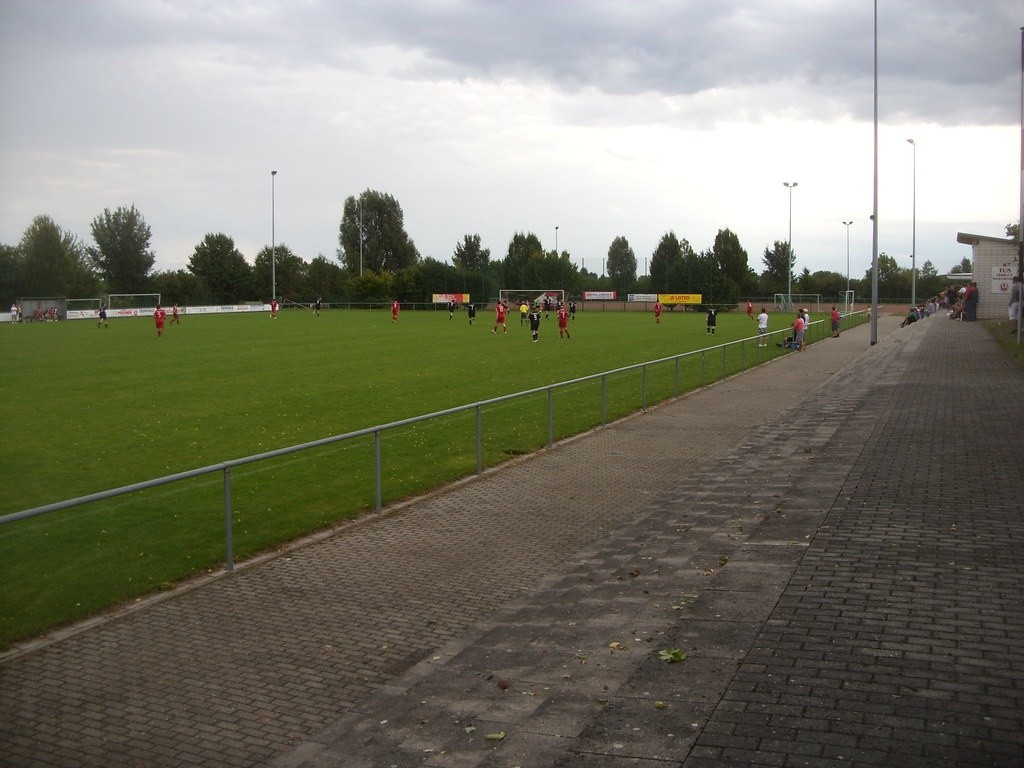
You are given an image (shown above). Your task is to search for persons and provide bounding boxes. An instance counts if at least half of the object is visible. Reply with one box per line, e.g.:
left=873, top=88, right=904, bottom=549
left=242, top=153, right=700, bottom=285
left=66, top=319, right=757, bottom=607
left=776, top=309, right=810, bottom=351
left=1008, top=276, right=1020, bottom=333
left=557, top=306, right=570, bottom=338
left=528, top=310, right=542, bottom=342
left=902, top=282, right=979, bottom=328
left=170, top=303, right=180, bottom=325
left=757, top=308, right=769, bottom=347
left=448, top=300, right=456, bottom=320
left=11, top=303, right=61, bottom=325
left=153, top=305, right=167, bottom=338
left=97, top=303, right=108, bottom=328
left=706, top=305, right=717, bottom=335
left=652, top=300, right=663, bottom=323
left=313, top=297, right=322, bottom=317
left=829, top=307, right=841, bottom=338
left=501, top=295, right=577, bottom=325
left=270, top=299, right=278, bottom=319
left=492, top=301, right=507, bottom=334
left=747, top=300, right=754, bottom=320
left=390, top=300, right=400, bottom=323
left=865, top=305, right=871, bottom=322
left=465, top=302, right=477, bottom=325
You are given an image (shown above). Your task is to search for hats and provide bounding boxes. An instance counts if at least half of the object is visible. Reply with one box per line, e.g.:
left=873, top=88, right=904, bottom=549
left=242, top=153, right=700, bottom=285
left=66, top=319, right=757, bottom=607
left=802, top=309, right=808, bottom=314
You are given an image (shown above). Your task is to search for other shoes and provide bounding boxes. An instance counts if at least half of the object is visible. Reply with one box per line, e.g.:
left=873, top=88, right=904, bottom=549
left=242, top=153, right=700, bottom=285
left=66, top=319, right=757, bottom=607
left=1010, top=329, right=1017, bottom=334
left=759, top=344, right=762, bottom=346
left=763, top=344, right=767, bottom=347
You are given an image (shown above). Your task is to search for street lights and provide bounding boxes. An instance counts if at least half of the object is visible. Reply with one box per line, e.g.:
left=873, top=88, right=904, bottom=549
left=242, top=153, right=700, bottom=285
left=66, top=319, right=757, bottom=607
left=271, top=170, right=276, bottom=299
left=360, top=197, right=366, bottom=276
left=556, top=226, right=559, bottom=252
left=843, top=221, right=853, bottom=311
left=907, top=138, right=915, bottom=308
left=783, top=182, right=797, bottom=301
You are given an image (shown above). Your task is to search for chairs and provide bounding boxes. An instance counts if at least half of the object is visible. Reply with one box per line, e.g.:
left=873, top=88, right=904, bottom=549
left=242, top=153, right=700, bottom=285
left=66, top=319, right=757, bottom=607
left=960, top=311, right=966, bottom=320
